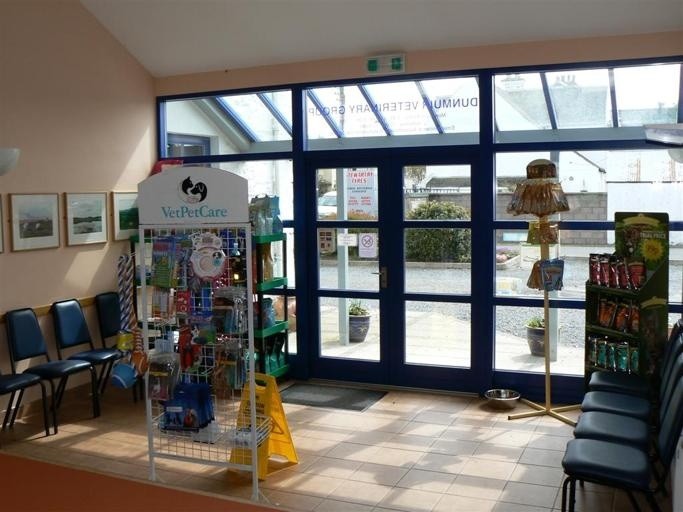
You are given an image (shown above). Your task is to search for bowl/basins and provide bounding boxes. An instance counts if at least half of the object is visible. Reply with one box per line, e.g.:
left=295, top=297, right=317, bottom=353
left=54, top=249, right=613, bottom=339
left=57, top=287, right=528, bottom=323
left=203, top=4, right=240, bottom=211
left=484, top=389, right=521, bottom=409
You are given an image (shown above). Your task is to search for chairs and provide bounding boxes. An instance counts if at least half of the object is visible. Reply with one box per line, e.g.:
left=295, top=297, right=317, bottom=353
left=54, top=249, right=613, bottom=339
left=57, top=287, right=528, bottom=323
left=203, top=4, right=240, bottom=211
left=559, top=320, right=682, bottom=510
left=0, top=291, right=165, bottom=436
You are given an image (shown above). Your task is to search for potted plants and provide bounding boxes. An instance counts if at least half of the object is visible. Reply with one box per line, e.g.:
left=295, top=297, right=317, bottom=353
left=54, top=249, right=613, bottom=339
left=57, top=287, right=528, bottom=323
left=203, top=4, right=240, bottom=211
left=347, top=302, right=370, bottom=342
left=524, top=316, right=545, bottom=356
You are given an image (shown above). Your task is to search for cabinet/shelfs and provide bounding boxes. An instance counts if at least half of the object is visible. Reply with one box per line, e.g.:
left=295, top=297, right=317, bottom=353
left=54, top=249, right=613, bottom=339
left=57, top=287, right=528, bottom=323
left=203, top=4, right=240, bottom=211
left=584, top=254, right=669, bottom=394
left=128, top=232, right=290, bottom=395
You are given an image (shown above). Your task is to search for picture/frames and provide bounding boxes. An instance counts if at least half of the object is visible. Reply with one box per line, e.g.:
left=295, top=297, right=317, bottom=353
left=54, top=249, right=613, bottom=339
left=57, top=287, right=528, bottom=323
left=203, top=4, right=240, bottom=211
left=0, top=192, right=151, bottom=253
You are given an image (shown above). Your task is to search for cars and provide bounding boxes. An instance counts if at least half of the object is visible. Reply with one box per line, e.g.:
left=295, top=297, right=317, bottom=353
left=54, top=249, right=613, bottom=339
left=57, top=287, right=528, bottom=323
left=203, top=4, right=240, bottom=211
left=316, top=190, right=337, bottom=241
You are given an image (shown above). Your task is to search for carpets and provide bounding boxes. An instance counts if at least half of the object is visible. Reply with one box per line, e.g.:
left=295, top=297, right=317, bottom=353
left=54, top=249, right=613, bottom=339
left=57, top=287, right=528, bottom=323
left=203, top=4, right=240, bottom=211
left=278, top=382, right=389, bottom=414
